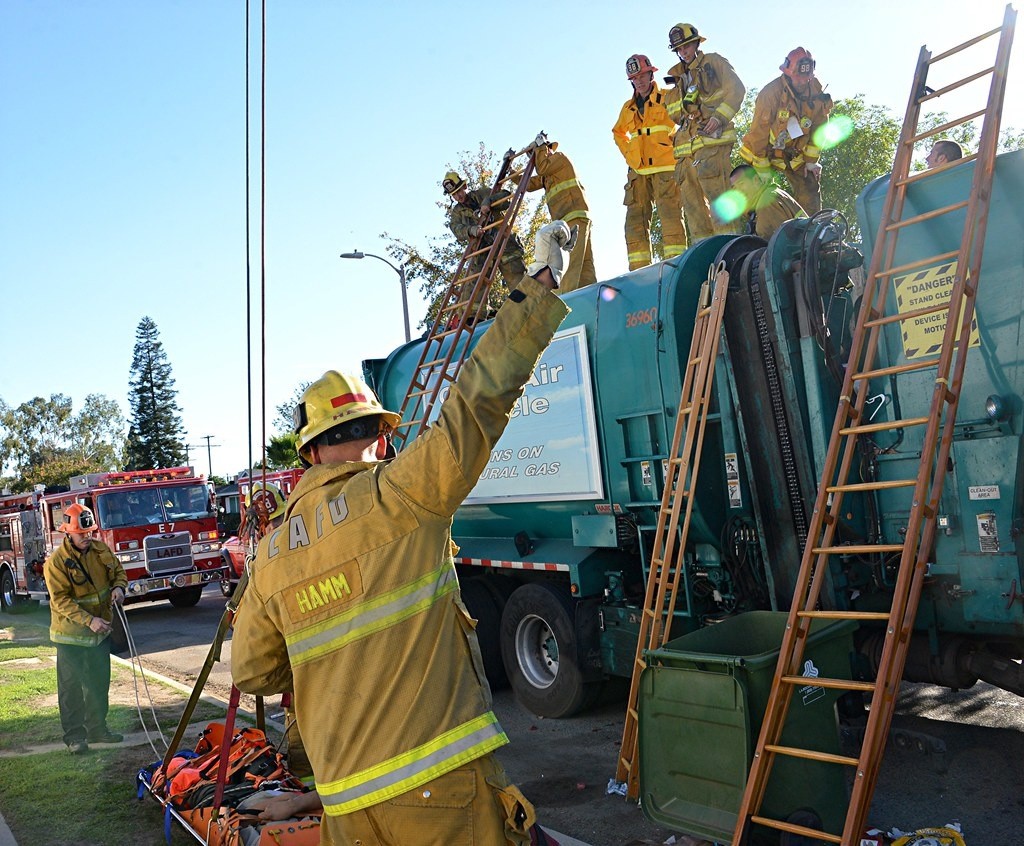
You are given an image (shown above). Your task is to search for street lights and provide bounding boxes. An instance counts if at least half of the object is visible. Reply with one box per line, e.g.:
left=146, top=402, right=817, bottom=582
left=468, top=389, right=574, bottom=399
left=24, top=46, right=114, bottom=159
left=341, top=251, right=410, bottom=343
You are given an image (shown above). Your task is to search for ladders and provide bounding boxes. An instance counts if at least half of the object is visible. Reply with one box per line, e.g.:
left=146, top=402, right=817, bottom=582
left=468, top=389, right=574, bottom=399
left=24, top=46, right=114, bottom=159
left=390, top=142, right=546, bottom=453
left=616, top=269, right=727, bottom=803
left=730, top=2, right=1018, bottom=845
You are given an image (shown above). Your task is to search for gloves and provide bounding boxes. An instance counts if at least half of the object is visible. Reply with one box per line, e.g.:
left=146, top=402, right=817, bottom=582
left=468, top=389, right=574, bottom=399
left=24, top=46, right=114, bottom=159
left=535, top=134, right=547, bottom=146
left=503, top=151, right=516, bottom=173
left=527, top=219, right=579, bottom=288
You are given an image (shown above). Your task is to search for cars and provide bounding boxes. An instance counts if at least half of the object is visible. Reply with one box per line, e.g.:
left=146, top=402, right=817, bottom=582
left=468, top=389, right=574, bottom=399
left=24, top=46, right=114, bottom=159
left=218, top=531, right=248, bottom=596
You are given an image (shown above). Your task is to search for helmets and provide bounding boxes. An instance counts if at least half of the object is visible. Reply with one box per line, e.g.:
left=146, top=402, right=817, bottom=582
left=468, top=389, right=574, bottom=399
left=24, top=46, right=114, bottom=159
left=525, top=139, right=558, bottom=165
left=626, top=53, right=658, bottom=80
left=59, top=503, right=98, bottom=534
left=668, top=22, right=706, bottom=52
left=442, top=171, right=468, bottom=196
left=245, top=481, right=288, bottom=521
left=779, top=46, right=816, bottom=80
left=293, top=369, right=402, bottom=468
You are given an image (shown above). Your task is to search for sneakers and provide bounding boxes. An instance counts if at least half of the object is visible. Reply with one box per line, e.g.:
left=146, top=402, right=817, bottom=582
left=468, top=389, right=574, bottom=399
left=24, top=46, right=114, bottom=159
left=85, top=728, right=125, bottom=742
left=68, top=738, right=88, bottom=754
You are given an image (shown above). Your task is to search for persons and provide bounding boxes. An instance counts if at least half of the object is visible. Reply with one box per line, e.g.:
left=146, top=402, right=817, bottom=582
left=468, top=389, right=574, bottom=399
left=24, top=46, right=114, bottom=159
left=924, top=140, right=963, bottom=170
left=244, top=481, right=288, bottom=537
left=729, top=164, right=811, bottom=243
left=442, top=168, right=528, bottom=327
left=662, top=21, right=746, bottom=247
left=611, top=53, right=687, bottom=272
left=502, top=133, right=599, bottom=293
left=227, top=218, right=576, bottom=845
left=41, top=502, right=129, bottom=756
left=743, top=44, right=835, bottom=218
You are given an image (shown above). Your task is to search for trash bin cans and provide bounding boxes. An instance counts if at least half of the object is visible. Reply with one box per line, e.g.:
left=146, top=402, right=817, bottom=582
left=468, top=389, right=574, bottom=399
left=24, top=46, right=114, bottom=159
left=635, top=610, right=859, bottom=846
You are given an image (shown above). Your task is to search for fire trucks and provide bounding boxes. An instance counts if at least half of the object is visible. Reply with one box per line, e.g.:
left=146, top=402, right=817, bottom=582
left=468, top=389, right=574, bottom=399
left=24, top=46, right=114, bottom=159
left=238, top=468, right=306, bottom=527
left=0, top=467, right=229, bottom=611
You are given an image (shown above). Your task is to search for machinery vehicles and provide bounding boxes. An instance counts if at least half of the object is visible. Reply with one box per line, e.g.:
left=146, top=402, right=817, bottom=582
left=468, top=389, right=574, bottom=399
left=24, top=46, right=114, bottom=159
left=377, top=146, right=1023, bottom=722
